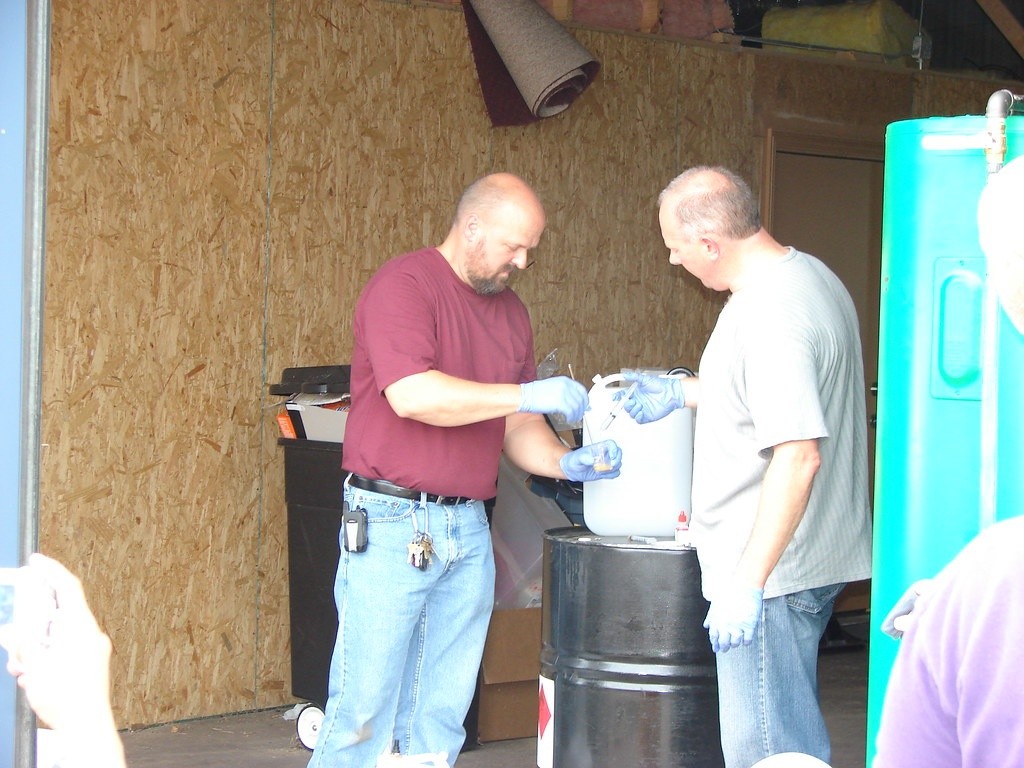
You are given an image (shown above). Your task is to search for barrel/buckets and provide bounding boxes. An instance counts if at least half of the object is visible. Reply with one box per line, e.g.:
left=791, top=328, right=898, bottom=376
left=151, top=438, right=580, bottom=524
left=535, top=527, right=724, bottom=767
left=582, top=373, right=694, bottom=536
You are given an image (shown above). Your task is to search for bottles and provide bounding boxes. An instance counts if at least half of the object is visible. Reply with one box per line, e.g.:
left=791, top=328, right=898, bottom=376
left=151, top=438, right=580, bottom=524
left=675, top=510, right=691, bottom=546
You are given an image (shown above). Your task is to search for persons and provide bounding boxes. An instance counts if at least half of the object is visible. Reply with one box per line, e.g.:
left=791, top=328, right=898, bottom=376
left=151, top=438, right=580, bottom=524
left=305, top=172, right=622, bottom=768
left=871, top=515, right=1024, bottom=768
left=611, top=165, right=871, bottom=768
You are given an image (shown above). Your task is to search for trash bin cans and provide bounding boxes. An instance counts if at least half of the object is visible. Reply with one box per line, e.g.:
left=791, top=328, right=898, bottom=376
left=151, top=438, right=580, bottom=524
left=269, top=364, right=481, bottom=755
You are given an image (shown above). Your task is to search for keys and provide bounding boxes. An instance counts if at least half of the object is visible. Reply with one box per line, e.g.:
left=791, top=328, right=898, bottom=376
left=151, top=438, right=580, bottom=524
left=407, top=531, right=440, bottom=568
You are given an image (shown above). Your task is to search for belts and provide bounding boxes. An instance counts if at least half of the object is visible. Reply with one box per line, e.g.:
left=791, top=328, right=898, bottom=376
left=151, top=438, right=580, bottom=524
left=348, top=474, right=470, bottom=505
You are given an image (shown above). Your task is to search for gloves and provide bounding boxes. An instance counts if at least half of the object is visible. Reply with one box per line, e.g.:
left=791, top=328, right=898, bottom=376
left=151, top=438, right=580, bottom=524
left=703, top=582, right=764, bottom=652
left=881, top=579, right=933, bottom=640
left=612, top=372, right=684, bottom=424
left=516, top=375, right=591, bottom=425
left=560, top=440, right=622, bottom=482
